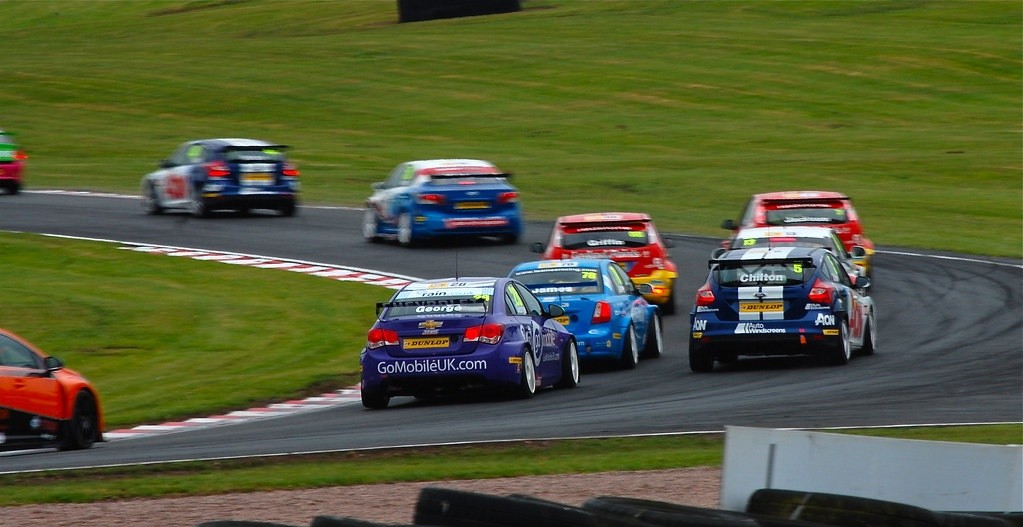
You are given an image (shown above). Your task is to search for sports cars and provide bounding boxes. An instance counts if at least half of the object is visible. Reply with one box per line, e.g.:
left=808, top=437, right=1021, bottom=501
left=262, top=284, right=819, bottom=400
left=0, top=124, right=881, bottom=451
